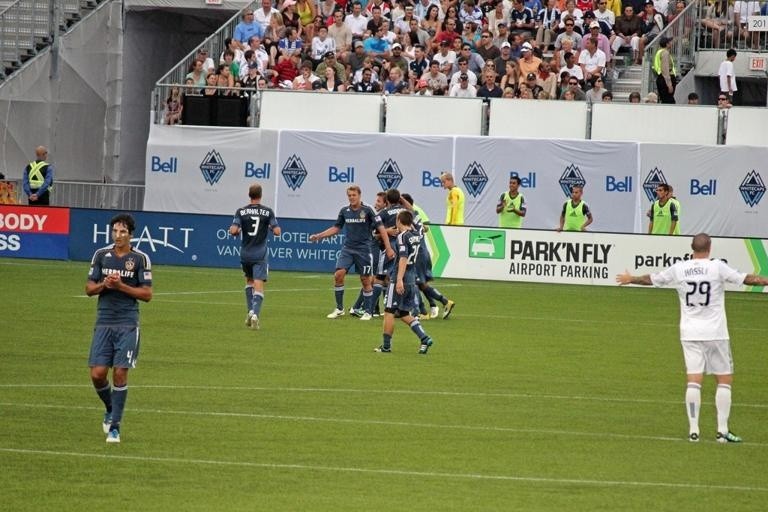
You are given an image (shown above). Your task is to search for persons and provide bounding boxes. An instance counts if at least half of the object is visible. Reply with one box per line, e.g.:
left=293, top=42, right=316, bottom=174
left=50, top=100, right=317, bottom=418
left=161, top=0, right=767, bottom=128
left=496, top=176, right=527, bottom=228
left=558, top=186, right=593, bottom=232
left=648, top=183, right=681, bottom=235
left=617, top=231, right=768, bottom=444
left=84, top=212, right=153, bottom=446
left=230, top=183, right=282, bottom=332
left=375, top=211, right=435, bottom=354
left=310, top=185, right=456, bottom=322
left=440, top=173, right=464, bottom=225
left=21, top=144, right=55, bottom=206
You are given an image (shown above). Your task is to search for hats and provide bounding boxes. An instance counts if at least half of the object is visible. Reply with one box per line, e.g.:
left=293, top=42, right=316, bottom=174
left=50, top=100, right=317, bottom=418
left=660, top=37, right=671, bottom=46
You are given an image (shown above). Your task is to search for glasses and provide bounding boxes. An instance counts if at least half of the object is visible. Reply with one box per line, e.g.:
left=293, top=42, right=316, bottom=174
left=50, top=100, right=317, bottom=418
left=717, top=97, right=729, bottom=101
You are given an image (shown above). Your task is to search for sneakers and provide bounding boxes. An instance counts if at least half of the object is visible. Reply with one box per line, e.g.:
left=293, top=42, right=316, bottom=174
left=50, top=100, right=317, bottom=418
left=687, top=434, right=700, bottom=442
left=371, top=345, right=390, bottom=352
left=327, top=299, right=454, bottom=320
left=245, top=310, right=259, bottom=329
left=716, top=431, right=742, bottom=443
left=416, top=336, right=433, bottom=353
left=104, top=407, right=120, bottom=442
